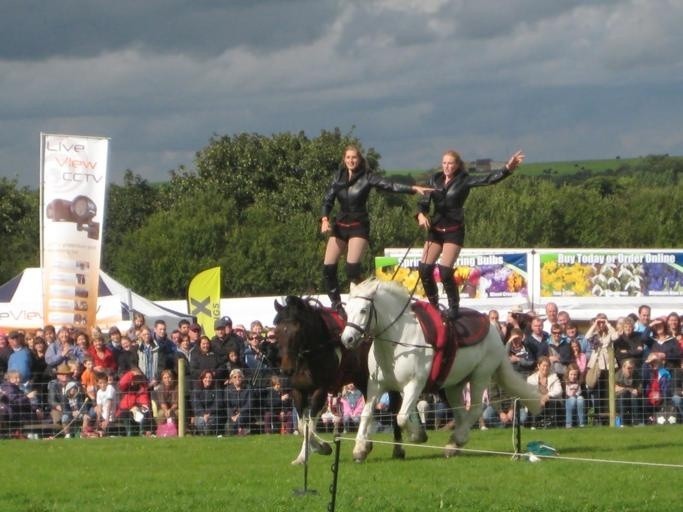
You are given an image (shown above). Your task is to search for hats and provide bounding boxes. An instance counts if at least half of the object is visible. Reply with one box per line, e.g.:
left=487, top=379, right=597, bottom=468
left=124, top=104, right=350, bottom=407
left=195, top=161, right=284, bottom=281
left=8, top=316, right=246, bottom=381
left=505, top=305, right=544, bottom=321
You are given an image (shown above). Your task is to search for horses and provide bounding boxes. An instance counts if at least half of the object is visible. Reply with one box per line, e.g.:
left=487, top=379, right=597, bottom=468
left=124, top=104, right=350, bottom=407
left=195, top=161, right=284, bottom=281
left=273, top=294, right=427, bottom=466
left=340, top=274, right=542, bottom=464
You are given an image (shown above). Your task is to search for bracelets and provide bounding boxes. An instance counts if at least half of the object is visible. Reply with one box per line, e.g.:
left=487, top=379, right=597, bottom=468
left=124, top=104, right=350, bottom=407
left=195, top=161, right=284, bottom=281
left=320, top=216, right=327, bottom=223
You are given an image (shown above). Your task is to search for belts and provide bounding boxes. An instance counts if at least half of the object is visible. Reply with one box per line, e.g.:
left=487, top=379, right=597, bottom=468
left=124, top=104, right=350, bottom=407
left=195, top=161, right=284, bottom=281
left=434, top=224, right=463, bottom=233
left=335, top=219, right=361, bottom=228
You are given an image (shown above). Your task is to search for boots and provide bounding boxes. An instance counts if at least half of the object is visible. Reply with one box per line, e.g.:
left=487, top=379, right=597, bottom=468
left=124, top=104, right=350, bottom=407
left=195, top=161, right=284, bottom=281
left=419, top=261, right=439, bottom=311
left=323, top=264, right=346, bottom=318
left=345, top=261, right=362, bottom=292
left=438, top=264, right=460, bottom=319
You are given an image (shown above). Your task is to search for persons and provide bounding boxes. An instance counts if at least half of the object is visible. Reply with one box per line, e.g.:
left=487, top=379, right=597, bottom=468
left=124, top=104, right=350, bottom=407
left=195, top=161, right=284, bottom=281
left=488, top=302, right=683, bottom=430
left=413, top=149, right=527, bottom=323
left=319, top=143, right=435, bottom=322
left=315, top=382, right=497, bottom=434
left=1, top=312, right=300, bottom=439
